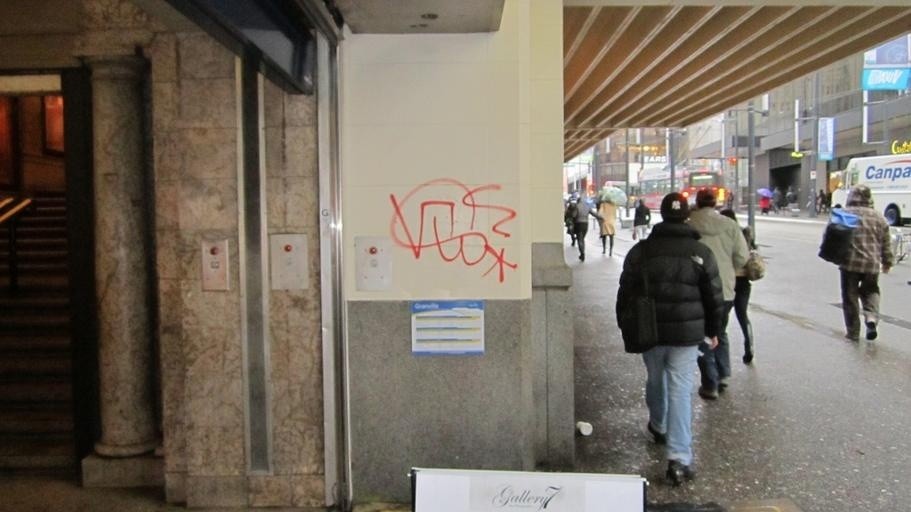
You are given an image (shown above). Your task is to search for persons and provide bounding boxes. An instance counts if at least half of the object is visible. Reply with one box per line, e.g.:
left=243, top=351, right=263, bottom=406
left=615, top=189, right=727, bottom=488
left=817, top=189, right=827, bottom=214
left=773, top=187, right=784, bottom=213
left=786, top=186, right=799, bottom=203
left=825, top=182, right=896, bottom=344
left=761, top=194, right=771, bottom=215
left=719, top=207, right=765, bottom=364
left=692, top=182, right=752, bottom=401
left=564, top=187, right=652, bottom=262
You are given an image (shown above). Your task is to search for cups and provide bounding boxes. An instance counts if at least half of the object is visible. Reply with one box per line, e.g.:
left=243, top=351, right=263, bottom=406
left=576, top=421, right=592, bottom=437
left=696, top=337, right=712, bottom=358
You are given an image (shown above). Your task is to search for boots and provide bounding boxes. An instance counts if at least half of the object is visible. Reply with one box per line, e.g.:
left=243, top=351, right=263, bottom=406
left=743, top=323, right=754, bottom=364
left=602, top=246, right=613, bottom=256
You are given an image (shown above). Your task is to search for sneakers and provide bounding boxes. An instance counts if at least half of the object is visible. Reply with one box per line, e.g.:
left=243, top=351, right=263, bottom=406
left=844, top=331, right=859, bottom=340
left=647, top=420, right=667, bottom=444
left=865, top=316, right=878, bottom=340
left=699, top=385, right=719, bottom=399
left=665, top=460, right=695, bottom=486
left=718, top=375, right=730, bottom=387
left=578, top=252, right=584, bottom=261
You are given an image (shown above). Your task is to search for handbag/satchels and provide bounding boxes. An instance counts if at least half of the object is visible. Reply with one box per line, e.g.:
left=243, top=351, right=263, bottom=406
left=743, top=229, right=767, bottom=281
left=818, top=206, right=859, bottom=266
left=632, top=225, right=637, bottom=240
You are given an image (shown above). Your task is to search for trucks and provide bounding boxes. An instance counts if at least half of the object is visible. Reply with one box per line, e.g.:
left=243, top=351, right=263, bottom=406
left=831, top=154, right=911, bottom=226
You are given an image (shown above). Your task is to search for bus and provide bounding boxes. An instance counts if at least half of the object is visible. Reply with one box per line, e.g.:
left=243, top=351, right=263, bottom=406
left=638, top=167, right=725, bottom=214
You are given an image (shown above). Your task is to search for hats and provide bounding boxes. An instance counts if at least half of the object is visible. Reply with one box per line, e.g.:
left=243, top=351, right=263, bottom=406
left=660, top=192, right=691, bottom=219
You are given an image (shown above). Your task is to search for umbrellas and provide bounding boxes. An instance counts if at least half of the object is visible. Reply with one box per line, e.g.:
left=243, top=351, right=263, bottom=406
left=756, top=187, right=775, bottom=198
